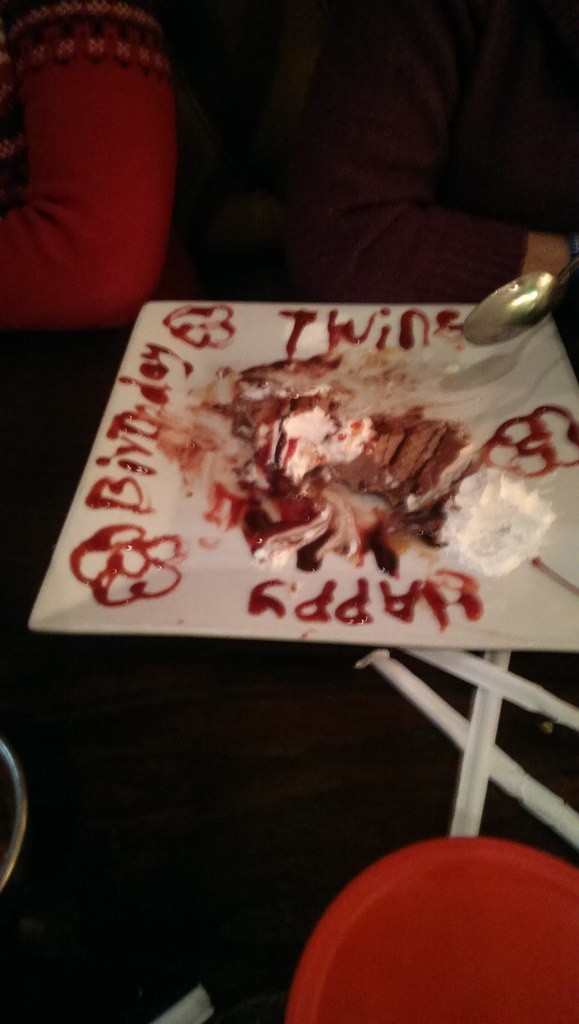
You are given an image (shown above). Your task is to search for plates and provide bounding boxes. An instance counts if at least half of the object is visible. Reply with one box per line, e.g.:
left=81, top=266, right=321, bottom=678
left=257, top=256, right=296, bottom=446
left=25, top=300, right=579, bottom=655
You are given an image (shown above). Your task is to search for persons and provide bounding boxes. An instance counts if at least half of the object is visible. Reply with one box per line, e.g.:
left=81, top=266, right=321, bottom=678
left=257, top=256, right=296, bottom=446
left=285, top=0, right=579, bottom=303
left=0, top=0, right=177, bottom=330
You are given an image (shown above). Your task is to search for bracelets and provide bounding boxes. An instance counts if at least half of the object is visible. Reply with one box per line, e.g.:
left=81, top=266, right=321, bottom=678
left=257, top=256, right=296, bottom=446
left=567, top=233, right=579, bottom=277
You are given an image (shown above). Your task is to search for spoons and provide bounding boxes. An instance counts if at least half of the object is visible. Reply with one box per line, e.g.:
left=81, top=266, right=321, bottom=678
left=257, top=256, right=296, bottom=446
left=462, top=254, right=579, bottom=345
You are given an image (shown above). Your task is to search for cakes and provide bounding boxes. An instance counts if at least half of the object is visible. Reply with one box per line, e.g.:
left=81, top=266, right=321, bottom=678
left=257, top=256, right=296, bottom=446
left=213, top=390, right=556, bottom=575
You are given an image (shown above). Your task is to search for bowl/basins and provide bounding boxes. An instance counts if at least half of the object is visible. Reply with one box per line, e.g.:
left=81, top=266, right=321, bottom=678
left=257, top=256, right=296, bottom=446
left=283, top=835, right=579, bottom=1024
